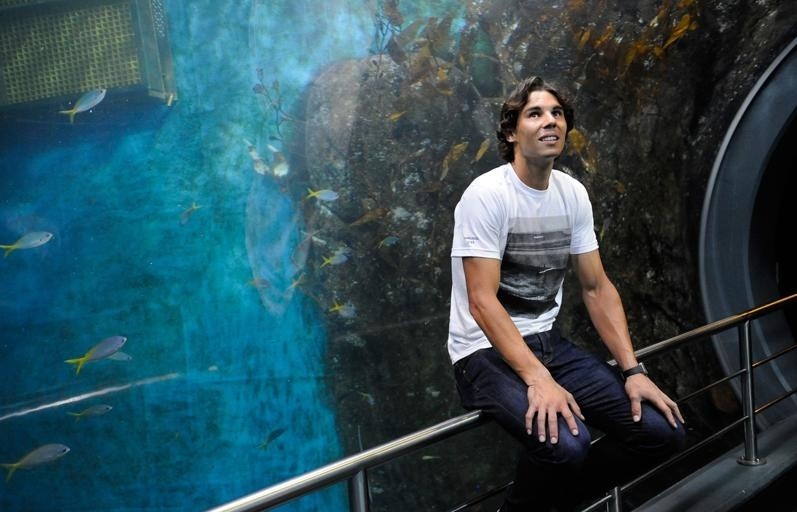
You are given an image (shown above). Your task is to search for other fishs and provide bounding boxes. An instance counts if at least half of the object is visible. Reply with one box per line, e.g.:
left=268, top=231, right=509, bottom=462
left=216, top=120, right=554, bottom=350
left=291, top=0, right=736, bottom=462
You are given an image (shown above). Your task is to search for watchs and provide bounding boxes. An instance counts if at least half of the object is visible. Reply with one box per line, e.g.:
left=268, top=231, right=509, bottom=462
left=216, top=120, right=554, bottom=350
left=620, top=361, right=649, bottom=381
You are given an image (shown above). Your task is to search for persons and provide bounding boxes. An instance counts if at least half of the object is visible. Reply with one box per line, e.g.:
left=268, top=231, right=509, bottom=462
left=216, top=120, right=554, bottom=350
left=444, top=75, right=687, bottom=511
